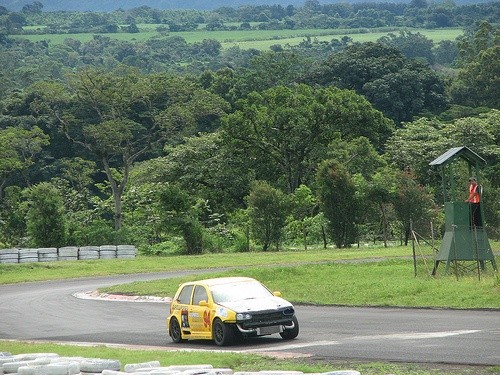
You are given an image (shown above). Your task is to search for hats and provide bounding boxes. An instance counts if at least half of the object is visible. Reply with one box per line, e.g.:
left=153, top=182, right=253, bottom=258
left=468, top=177, right=477, bottom=181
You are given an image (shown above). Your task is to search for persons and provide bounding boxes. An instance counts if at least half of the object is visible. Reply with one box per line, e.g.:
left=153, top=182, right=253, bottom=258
left=465, top=177, right=480, bottom=230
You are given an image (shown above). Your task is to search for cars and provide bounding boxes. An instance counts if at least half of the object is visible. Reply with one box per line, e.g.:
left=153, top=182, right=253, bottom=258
left=166, top=276, right=299, bottom=345
